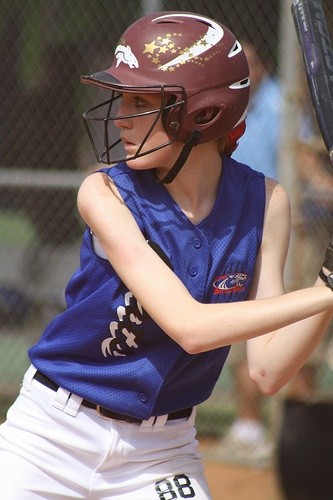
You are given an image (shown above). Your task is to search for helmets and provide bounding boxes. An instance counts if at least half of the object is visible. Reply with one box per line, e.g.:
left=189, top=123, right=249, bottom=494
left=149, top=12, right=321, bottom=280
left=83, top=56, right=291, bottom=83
left=81, top=12, right=250, bottom=145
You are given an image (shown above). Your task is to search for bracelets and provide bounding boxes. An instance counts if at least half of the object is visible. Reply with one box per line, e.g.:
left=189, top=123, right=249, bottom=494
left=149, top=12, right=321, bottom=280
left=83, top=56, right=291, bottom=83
left=318, top=267, right=330, bottom=285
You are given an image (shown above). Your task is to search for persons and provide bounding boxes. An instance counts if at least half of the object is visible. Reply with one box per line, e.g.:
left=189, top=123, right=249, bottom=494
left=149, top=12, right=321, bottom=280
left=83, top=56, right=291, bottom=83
left=0, top=10, right=333, bottom=500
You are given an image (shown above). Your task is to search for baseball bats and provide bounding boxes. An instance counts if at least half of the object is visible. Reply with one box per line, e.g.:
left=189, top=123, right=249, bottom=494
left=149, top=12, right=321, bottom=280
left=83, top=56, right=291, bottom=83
left=290, top=0, right=332, bottom=162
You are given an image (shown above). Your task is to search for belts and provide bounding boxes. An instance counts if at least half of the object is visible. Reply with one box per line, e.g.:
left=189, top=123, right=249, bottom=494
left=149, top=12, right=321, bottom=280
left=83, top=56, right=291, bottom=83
left=33, top=367, right=193, bottom=424
